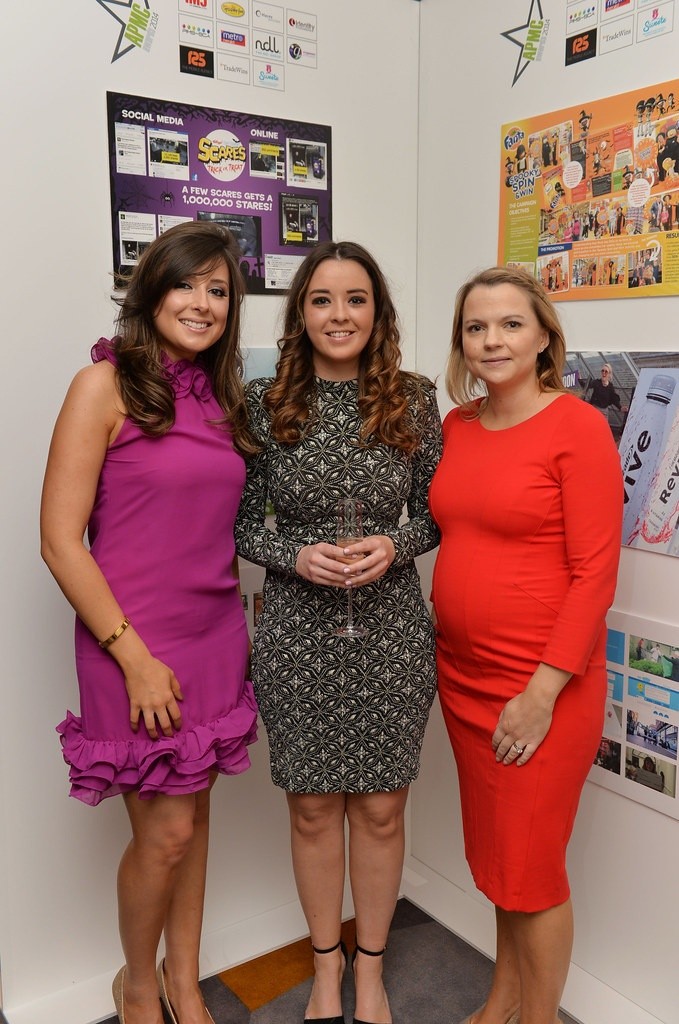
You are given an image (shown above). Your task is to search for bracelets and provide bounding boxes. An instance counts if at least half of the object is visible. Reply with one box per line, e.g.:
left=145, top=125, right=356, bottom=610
left=98, top=616, right=131, bottom=649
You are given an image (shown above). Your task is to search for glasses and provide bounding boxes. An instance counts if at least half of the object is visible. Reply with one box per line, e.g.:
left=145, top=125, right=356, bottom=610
left=600, top=369, right=610, bottom=373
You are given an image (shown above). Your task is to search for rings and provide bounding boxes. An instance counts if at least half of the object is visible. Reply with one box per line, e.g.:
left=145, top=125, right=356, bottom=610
left=513, top=743, right=523, bottom=753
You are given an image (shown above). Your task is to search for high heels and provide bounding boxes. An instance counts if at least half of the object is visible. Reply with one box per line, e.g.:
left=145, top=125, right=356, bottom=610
left=352, top=936, right=387, bottom=1024
left=112, top=964, right=158, bottom=1024
left=304, top=938, right=349, bottom=1024
left=156, top=957, right=215, bottom=1024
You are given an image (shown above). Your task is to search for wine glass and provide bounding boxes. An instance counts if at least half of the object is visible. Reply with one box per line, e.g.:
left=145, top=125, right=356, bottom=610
left=331, top=497, right=372, bottom=639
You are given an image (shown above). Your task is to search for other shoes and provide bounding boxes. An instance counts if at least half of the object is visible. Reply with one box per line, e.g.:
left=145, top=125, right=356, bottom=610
left=459, top=1001, right=521, bottom=1024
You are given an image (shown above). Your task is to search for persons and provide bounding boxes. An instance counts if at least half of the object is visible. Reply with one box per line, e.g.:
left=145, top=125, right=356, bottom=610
left=578, top=363, right=628, bottom=420
left=40, top=220, right=260, bottom=1024
left=428, top=267, right=624, bottom=1024
left=562, top=210, right=599, bottom=241
left=650, top=195, right=673, bottom=230
left=233, top=241, right=444, bottom=1024
left=637, top=251, right=658, bottom=283
left=616, top=207, right=625, bottom=235
